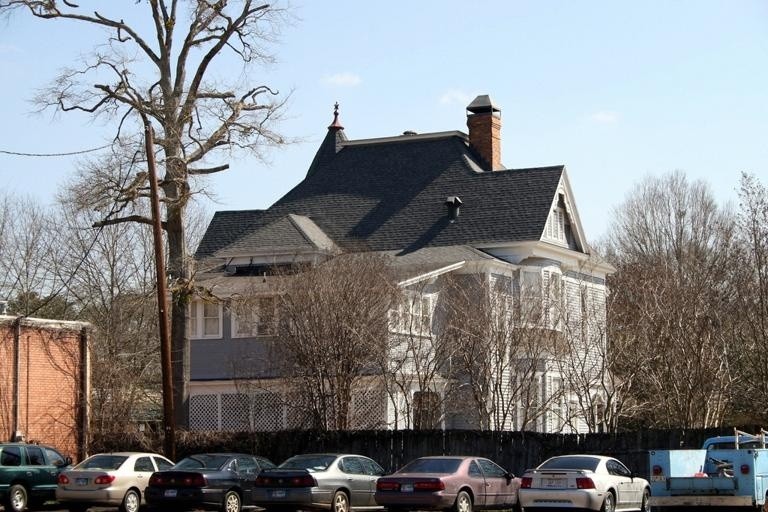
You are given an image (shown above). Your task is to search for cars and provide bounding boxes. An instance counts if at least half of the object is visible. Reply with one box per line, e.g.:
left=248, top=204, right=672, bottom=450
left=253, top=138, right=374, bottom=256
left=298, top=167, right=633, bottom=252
left=55, top=451, right=177, bottom=512
left=0, top=441, right=74, bottom=510
left=144, top=453, right=278, bottom=512
left=251, top=452, right=385, bottom=512
left=518, top=454, right=650, bottom=511
left=374, top=455, right=521, bottom=512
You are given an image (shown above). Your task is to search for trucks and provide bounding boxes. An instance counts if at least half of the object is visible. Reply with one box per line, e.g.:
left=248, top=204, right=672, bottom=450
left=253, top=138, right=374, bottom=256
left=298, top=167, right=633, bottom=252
left=648, top=432, right=767, bottom=512
